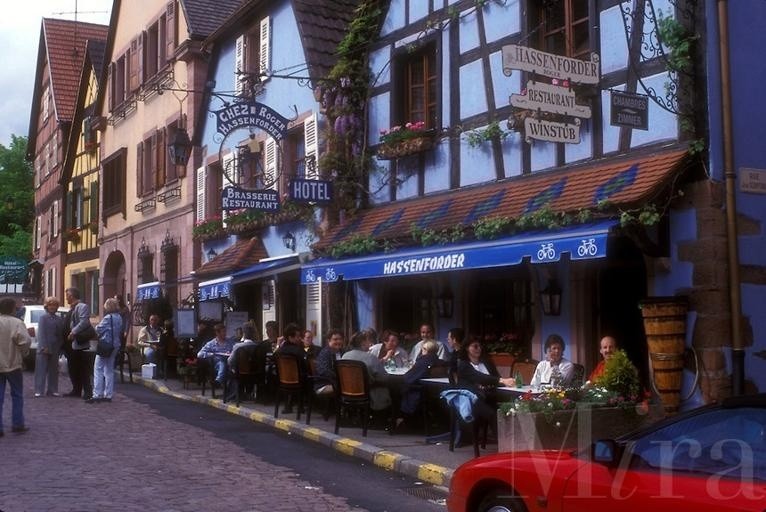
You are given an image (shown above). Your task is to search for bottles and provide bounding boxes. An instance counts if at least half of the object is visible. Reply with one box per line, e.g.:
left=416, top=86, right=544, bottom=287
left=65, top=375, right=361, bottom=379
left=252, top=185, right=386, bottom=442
left=515, top=369, right=523, bottom=389
left=535, top=368, right=542, bottom=387
left=385, top=357, right=414, bottom=372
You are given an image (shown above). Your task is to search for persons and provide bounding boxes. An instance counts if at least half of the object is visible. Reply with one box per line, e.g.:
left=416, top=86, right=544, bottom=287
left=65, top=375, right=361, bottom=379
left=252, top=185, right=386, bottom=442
left=64, top=287, right=95, bottom=399
left=156, top=319, right=179, bottom=379
left=197, top=322, right=518, bottom=427
left=85, top=297, right=122, bottom=403
left=530, top=334, right=574, bottom=388
left=235, top=132, right=263, bottom=177
left=0, top=298, right=31, bottom=438
left=138, top=314, right=161, bottom=364
left=114, top=294, right=131, bottom=369
left=587, top=336, right=623, bottom=466
left=34, top=296, right=66, bottom=397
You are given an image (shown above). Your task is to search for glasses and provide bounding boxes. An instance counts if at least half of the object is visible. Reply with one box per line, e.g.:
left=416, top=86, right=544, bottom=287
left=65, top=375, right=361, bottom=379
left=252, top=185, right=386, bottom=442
left=470, top=343, right=482, bottom=349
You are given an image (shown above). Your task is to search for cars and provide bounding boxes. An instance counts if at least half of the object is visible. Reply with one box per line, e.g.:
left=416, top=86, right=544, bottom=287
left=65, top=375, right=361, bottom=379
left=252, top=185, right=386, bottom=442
left=12, top=305, right=77, bottom=366
left=443, top=393, right=765, bottom=511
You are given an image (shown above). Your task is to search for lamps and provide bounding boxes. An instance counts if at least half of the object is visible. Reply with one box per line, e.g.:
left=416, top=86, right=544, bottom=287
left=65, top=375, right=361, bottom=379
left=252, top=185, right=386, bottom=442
left=435, top=286, right=454, bottom=317
left=282, top=231, right=294, bottom=247
left=207, top=248, right=217, bottom=261
left=167, top=90, right=192, bottom=165
left=538, top=278, right=562, bottom=317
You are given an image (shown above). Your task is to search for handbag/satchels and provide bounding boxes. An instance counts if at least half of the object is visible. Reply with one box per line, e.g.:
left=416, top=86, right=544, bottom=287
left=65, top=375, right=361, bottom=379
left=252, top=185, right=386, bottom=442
left=96, top=339, right=115, bottom=358
left=73, top=305, right=96, bottom=346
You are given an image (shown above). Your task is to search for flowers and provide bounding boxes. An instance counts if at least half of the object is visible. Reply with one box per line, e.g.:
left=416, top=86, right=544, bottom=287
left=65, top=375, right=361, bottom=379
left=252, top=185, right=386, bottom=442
left=378, top=122, right=427, bottom=144
left=519, top=77, right=568, bottom=94
left=499, top=348, right=648, bottom=416
left=193, top=194, right=307, bottom=242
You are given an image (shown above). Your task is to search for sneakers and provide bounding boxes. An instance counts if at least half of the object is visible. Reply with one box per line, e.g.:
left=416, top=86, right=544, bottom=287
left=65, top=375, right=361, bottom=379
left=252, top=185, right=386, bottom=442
left=34, top=391, right=113, bottom=404
left=10, top=425, right=31, bottom=433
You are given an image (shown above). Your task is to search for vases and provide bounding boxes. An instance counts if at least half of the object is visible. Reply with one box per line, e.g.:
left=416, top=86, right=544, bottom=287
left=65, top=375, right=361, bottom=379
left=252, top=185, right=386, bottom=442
left=514, top=95, right=590, bottom=130
left=376, top=136, right=430, bottom=160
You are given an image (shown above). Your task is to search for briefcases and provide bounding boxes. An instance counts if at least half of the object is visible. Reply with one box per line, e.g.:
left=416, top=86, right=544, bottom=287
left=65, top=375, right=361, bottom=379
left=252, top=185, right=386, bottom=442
left=140, top=362, right=158, bottom=379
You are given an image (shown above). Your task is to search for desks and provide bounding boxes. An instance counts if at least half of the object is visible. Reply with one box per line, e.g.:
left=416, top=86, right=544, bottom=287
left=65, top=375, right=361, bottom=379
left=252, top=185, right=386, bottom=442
left=420, top=367, right=566, bottom=409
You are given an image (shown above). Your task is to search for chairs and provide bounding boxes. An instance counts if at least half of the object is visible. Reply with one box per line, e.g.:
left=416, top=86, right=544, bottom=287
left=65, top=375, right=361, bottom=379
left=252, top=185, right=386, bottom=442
left=508, top=358, right=536, bottom=385
left=200, top=335, right=433, bottom=439
left=126, top=333, right=194, bottom=398
left=442, top=367, right=492, bottom=458
